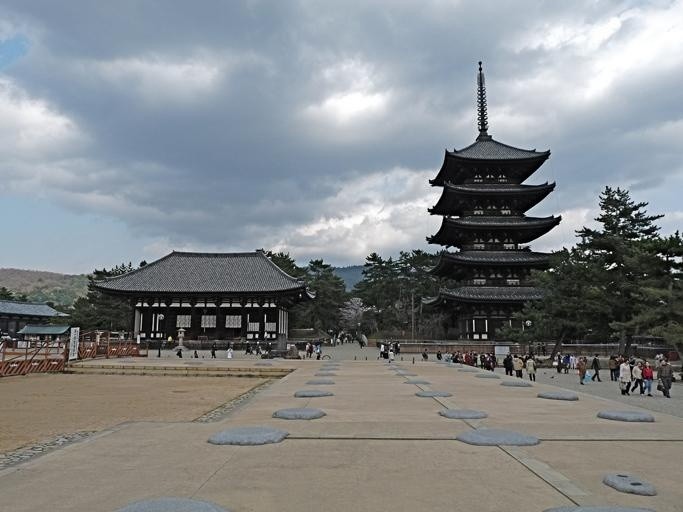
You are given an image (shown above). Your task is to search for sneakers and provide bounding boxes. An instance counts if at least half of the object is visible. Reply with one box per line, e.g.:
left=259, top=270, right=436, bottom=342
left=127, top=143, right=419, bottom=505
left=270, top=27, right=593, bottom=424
left=667, top=396, right=670, bottom=398
left=627, top=389, right=653, bottom=397
left=592, top=378, right=601, bottom=382
left=663, top=392, right=666, bottom=396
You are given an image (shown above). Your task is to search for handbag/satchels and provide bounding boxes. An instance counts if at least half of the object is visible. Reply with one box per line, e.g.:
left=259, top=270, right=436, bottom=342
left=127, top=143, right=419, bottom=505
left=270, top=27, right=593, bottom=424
left=657, top=385, right=665, bottom=391
left=618, top=383, right=626, bottom=391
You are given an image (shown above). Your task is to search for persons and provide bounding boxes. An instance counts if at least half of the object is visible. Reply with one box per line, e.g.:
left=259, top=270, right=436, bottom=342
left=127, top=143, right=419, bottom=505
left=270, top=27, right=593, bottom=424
left=423, top=348, right=428, bottom=360
left=379, top=339, right=400, bottom=361
left=437, top=341, right=672, bottom=399
left=304, top=341, right=321, bottom=359
left=162, top=336, right=271, bottom=359
left=330, top=328, right=364, bottom=349
